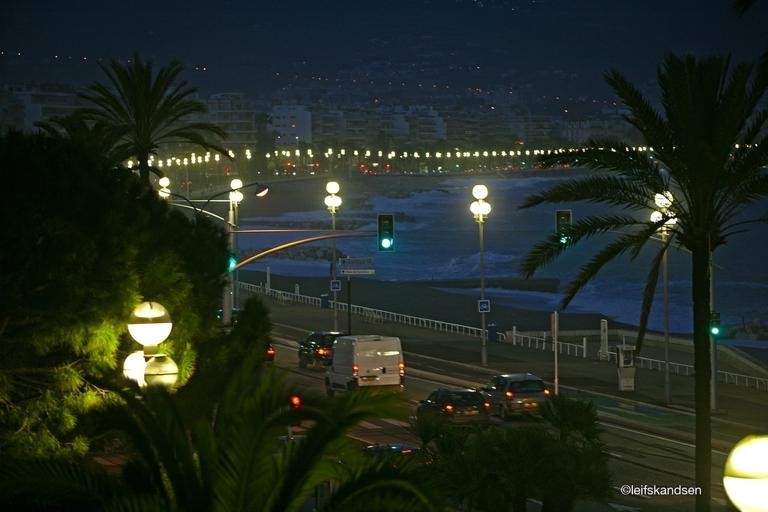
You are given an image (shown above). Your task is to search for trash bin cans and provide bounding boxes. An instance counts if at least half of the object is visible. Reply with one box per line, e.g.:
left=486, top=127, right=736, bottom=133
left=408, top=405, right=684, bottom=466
left=488, top=324, right=497, bottom=342
left=320, top=293, right=330, bottom=309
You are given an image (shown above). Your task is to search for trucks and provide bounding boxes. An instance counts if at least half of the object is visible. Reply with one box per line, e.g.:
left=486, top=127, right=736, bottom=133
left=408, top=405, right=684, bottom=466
left=321, top=335, right=407, bottom=400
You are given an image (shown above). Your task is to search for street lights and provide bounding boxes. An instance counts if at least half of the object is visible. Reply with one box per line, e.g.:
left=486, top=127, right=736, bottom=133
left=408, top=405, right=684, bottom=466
left=646, top=190, right=679, bottom=403
left=714, top=431, right=768, bottom=511
left=227, top=177, right=244, bottom=325
left=317, top=177, right=344, bottom=334
left=154, top=182, right=270, bottom=337
left=113, top=296, right=184, bottom=401
left=465, top=181, right=495, bottom=366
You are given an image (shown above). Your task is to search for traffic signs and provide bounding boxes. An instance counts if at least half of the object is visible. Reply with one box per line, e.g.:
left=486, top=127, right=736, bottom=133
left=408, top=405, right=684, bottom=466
left=338, top=257, right=375, bottom=266
left=340, top=269, right=375, bottom=276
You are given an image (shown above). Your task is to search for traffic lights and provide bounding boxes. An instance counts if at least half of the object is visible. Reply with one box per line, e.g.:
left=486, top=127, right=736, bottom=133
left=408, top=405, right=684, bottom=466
left=375, top=214, right=396, bottom=253
left=554, top=208, right=574, bottom=246
left=224, top=253, right=239, bottom=274
left=710, top=310, right=721, bottom=337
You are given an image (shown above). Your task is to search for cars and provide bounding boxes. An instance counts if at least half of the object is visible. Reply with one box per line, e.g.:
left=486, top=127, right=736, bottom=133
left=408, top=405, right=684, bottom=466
left=260, top=334, right=277, bottom=364
left=338, top=442, right=437, bottom=475
left=213, top=308, right=241, bottom=328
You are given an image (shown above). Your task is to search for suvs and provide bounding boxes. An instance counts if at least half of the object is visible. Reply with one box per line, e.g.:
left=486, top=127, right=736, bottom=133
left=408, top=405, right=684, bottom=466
left=295, top=330, right=345, bottom=369
left=478, top=372, right=551, bottom=420
left=413, top=386, right=494, bottom=437
